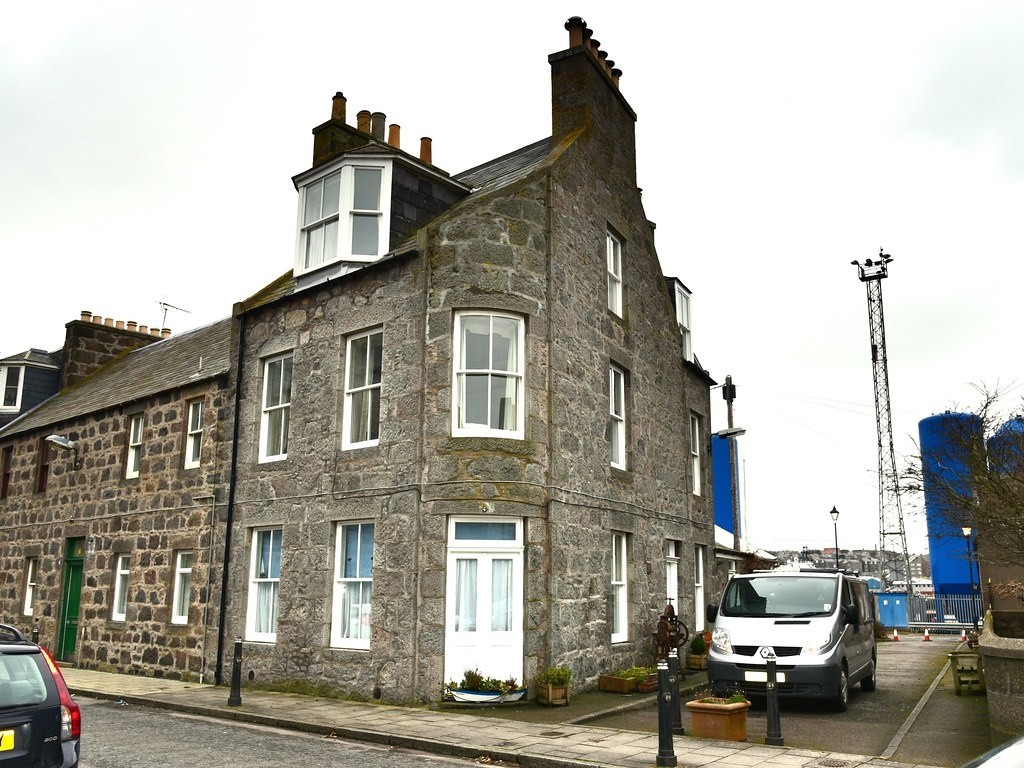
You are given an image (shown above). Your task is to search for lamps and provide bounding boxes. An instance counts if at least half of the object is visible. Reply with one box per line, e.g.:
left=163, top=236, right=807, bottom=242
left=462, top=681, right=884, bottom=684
left=45, top=434, right=77, bottom=467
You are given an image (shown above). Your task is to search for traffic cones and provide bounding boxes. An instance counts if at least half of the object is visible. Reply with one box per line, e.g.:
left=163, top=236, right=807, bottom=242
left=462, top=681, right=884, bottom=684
left=892, top=626, right=900, bottom=642
left=958, top=625, right=968, bottom=643
left=922, top=626, right=933, bottom=642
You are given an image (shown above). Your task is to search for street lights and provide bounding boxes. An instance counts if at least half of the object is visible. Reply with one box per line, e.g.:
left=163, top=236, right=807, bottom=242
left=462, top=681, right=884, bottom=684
left=959, top=520, right=979, bottom=635
left=829, top=506, right=840, bottom=569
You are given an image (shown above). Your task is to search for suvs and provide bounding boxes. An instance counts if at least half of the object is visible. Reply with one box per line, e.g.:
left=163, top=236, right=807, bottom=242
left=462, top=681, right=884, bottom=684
left=0, top=624, right=81, bottom=768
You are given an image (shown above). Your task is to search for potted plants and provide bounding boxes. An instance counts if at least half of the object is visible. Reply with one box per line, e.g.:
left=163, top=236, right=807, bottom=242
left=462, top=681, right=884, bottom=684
left=599, top=667, right=659, bottom=692
left=686, top=681, right=752, bottom=742
left=435, top=669, right=527, bottom=702
left=533, top=666, right=573, bottom=707
left=687, top=635, right=709, bottom=670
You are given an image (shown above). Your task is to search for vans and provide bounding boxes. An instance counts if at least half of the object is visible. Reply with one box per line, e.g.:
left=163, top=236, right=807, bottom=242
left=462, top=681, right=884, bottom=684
left=706, top=567, right=878, bottom=713
